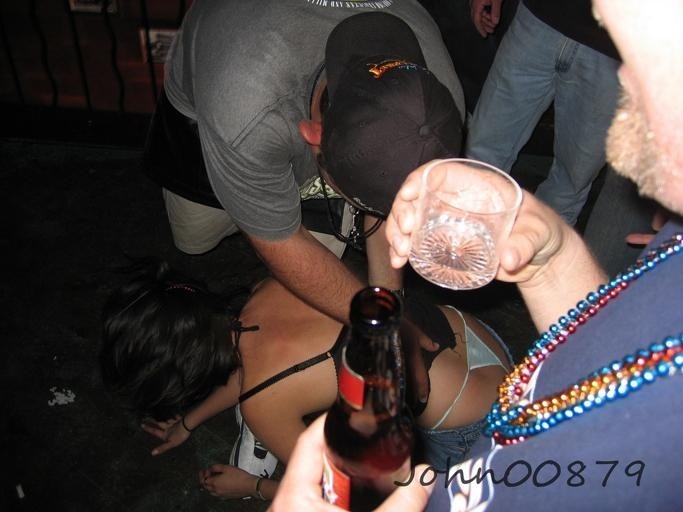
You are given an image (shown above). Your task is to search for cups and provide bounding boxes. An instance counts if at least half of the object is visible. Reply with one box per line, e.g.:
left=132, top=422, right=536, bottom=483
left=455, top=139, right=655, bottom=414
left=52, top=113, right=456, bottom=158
left=409, top=160, right=523, bottom=289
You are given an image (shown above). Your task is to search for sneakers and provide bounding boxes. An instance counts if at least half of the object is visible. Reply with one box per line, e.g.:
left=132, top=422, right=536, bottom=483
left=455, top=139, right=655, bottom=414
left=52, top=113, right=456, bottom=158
left=230, top=405, right=280, bottom=501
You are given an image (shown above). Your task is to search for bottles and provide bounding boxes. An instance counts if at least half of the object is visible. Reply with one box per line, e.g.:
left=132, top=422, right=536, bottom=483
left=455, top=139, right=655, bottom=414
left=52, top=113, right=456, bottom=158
left=323, top=285, right=416, bottom=512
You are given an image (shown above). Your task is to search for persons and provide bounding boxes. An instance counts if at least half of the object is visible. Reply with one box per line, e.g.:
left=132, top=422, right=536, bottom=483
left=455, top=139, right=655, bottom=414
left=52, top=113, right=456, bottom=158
left=266, top=0, right=683, bottom=512
left=105, top=278, right=516, bottom=503
left=161, top=0, right=465, bottom=352
left=463, top=1, right=626, bottom=228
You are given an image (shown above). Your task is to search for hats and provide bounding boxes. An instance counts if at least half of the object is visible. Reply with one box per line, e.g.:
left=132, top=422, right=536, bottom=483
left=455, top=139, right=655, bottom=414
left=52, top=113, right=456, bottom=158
left=319, top=12, right=467, bottom=226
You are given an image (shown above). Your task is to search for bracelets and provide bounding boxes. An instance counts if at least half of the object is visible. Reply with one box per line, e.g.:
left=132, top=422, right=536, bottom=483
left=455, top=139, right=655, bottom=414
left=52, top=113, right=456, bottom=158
left=181, top=416, right=194, bottom=433
left=255, top=476, right=268, bottom=502
